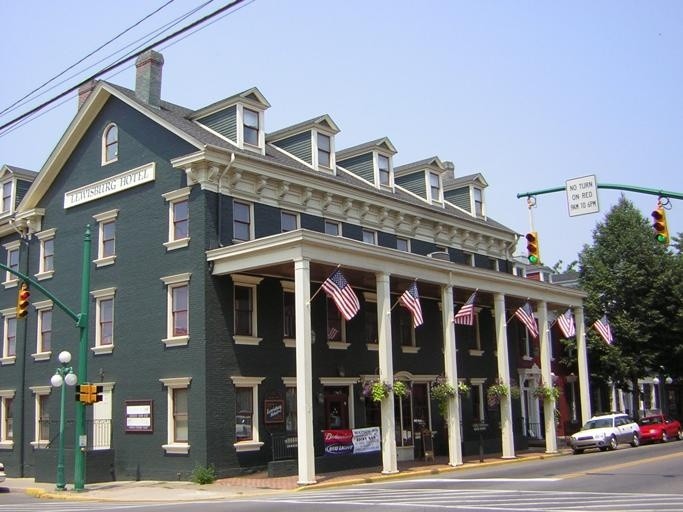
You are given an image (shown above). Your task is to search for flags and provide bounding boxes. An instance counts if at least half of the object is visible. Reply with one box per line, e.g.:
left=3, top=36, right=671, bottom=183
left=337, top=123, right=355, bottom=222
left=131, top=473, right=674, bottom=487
left=593, top=315, right=616, bottom=345
left=515, top=302, right=539, bottom=338
left=321, top=268, right=361, bottom=322
left=398, top=282, right=426, bottom=330
left=454, top=291, right=476, bottom=327
left=557, top=308, right=576, bottom=340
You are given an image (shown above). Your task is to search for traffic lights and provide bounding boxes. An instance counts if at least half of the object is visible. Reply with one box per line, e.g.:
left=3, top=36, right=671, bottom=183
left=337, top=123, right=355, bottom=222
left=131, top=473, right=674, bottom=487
left=91, top=385, right=104, bottom=404
left=650, top=208, right=671, bottom=247
left=75, top=383, right=91, bottom=407
left=526, top=232, right=540, bottom=265
left=17, top=290, right=31, bottom=319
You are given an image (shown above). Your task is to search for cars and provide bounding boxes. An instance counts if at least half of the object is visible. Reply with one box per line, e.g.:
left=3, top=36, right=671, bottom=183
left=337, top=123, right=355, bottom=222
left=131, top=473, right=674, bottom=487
left=0, top=462, right=7, bottom=486
left=568, top=411, right=643, bottom=454
left=636, top=414, right=682, bottom=444
left=234, top=407, right=254, bottom=440
left=394, top=414, right=428, bottom=446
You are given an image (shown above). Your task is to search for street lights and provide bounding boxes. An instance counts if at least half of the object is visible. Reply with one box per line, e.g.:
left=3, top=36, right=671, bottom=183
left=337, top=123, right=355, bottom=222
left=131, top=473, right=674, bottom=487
left=48, top=348, right=79, bottom=493
left=652, top=363, right=675, bottom=417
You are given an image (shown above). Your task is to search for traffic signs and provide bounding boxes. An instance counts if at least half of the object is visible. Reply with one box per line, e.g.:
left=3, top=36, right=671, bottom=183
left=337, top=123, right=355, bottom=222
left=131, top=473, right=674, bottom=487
left=564, top=174, right=600, bottom=218
